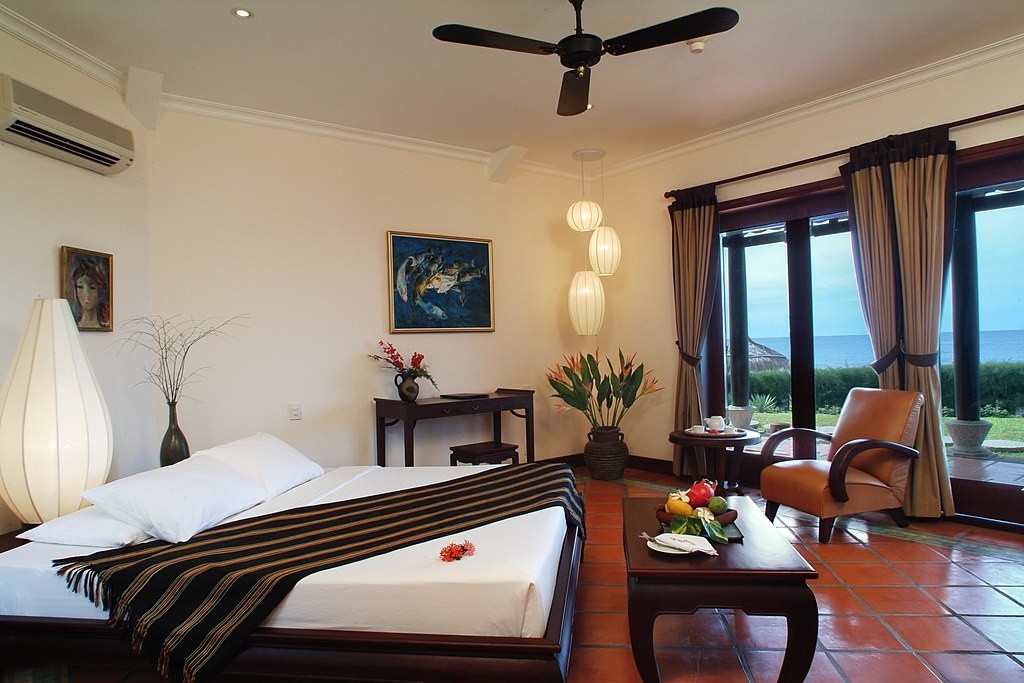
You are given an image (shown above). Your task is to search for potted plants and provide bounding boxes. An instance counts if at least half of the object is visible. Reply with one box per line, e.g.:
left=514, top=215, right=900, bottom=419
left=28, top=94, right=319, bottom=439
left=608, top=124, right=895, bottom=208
left=117, top=309, right=251, bottom=468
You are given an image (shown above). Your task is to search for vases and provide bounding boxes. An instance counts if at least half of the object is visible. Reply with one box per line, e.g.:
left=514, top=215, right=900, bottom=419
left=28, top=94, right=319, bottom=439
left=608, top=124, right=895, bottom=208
left=395, top=374, right=420, bottom=402
left=583, top=427, right=629, bottom=480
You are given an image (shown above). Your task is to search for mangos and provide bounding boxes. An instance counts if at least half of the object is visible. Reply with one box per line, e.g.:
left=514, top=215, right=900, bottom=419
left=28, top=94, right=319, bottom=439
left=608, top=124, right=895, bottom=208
left=667, top=499, right=692, bottom=515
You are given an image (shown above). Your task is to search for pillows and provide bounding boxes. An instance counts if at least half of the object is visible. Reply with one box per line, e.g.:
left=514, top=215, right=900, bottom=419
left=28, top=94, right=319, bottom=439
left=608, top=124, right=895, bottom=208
left=14, top=433, right=326, bottom=545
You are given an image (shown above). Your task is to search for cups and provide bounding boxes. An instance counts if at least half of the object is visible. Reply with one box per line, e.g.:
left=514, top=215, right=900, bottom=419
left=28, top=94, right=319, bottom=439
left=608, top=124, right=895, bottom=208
left=724, top=426, right=737, bottom=433
left=691, top=425, right=704, bottom=432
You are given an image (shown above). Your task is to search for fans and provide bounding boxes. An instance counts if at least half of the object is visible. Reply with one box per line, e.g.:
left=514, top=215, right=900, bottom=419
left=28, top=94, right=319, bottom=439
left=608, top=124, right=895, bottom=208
left=432, top=1, right=740, bottom=117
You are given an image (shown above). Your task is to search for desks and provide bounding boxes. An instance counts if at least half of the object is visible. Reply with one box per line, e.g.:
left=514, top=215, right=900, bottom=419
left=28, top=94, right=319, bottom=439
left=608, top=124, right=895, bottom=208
left=622, top=492, right=818, bottom=683
left=374, top=388, right=535, bottom=467
left=667, top=428, right=763, bottom=495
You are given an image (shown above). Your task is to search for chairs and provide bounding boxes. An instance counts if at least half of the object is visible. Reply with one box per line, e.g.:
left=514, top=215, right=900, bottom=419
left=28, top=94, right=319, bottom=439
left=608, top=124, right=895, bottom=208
left=758, top=387, right=925, bottom=544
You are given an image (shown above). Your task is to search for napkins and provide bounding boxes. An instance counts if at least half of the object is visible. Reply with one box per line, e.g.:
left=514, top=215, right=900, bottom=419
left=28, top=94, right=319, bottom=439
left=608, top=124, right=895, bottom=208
left=654, top=533, right=720, bottom=557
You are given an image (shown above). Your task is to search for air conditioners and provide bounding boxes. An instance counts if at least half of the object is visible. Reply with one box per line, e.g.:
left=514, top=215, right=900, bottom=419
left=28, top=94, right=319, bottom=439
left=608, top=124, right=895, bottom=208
left=1, top=71, right=135, bottom=177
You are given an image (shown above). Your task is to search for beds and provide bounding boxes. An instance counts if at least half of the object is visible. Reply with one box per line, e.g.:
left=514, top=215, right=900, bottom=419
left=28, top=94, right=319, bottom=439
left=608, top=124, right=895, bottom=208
left=1, top=460, right=584, bottom=683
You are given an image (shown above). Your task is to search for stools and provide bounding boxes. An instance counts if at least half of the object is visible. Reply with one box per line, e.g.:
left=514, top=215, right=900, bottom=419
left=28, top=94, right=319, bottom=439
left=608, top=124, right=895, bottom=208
left=449, top=440, right=520, bottom=467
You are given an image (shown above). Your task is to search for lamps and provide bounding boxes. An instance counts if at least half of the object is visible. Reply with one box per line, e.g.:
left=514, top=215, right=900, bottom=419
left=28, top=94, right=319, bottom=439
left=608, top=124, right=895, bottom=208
left=567, top=149, right=621, bottom=336
left=0, top=299, right=114, bottom=523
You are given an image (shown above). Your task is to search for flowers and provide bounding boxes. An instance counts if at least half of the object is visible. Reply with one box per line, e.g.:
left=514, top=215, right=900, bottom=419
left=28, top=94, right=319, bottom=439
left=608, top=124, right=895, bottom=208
left=546, top=346, right=666, bottom=429
left=367, top=340, right=441, bottom=392
left=439, top=539, right=476, bottom=562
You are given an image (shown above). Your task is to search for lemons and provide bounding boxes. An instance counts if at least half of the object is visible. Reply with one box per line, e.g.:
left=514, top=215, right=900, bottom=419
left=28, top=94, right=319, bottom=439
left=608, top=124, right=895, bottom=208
left=708, top=496, right=728, bottom=514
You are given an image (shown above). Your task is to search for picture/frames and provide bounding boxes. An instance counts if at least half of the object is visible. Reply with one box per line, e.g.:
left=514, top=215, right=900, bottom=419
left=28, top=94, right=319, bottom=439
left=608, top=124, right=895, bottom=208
left=60, top=245, right=114, bottom=333
left=387, top=228, right=495, bottom=335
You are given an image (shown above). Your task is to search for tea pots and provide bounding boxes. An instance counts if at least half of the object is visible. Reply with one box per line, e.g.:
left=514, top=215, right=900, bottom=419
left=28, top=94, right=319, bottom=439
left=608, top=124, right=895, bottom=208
left=704, top=415, right=730, bottom=429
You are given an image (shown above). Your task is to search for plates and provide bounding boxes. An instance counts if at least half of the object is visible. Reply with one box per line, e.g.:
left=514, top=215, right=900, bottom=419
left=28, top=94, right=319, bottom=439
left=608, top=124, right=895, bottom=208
left=647, top=536, right=689, bottom=553
left=706, top=427, right=724, bottom=431
